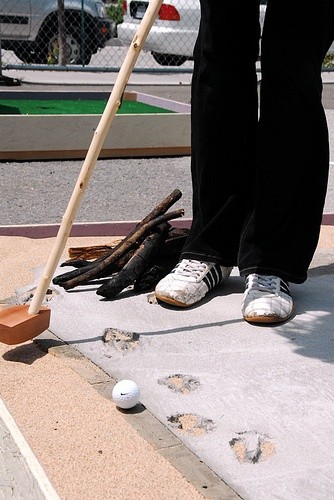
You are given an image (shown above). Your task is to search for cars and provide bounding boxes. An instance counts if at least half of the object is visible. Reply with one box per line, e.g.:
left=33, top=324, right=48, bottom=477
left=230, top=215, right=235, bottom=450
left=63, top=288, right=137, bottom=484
left=117, top=0, right=334, bottom=66
left=0, top=0, right=116, bottom=65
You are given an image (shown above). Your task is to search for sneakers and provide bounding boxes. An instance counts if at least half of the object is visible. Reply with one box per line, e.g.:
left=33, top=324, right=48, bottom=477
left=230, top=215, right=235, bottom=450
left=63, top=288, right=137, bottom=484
left=242, top=273, right=294, bottom=325
left=155, top=259, right=233, bottom=308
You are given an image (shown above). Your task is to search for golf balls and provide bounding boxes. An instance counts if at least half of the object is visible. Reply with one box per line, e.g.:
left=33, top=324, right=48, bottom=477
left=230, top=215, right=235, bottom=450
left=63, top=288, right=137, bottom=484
left=111, top=379, right=141, bottom=412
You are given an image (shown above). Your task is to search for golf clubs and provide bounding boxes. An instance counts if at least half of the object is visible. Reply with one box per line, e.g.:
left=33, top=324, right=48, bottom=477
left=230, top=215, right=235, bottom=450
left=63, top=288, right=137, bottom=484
left=0, top=1, right=163, bottom=345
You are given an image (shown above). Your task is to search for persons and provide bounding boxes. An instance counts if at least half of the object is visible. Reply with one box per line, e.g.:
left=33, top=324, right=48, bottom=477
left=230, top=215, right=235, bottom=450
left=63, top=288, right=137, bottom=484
left=154, top=0, right=334, bottom=324
